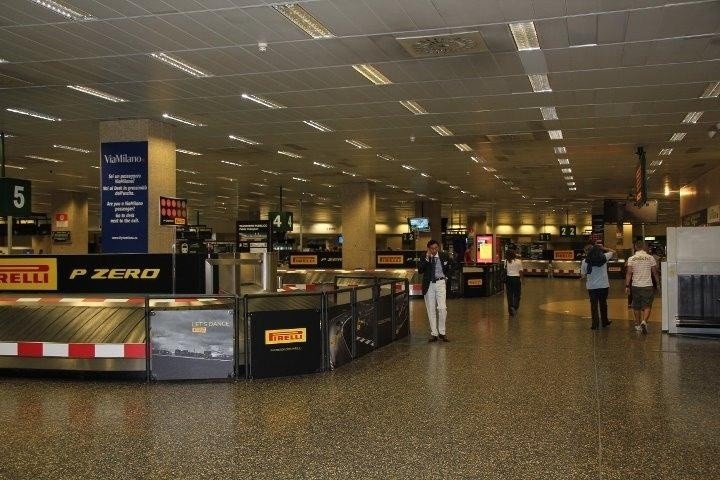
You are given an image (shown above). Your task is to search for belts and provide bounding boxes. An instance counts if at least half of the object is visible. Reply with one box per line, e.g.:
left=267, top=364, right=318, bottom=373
left=430, top=277, right=445, bottom=281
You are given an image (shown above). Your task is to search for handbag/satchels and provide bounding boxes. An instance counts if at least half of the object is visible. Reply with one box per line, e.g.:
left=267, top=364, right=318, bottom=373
left=498, top=269, right=507, bottom=283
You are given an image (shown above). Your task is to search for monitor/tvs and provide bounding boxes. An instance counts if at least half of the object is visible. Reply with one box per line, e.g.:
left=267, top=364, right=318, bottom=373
left=407, top=216, right=431, bottom=233
left=337, top=235, right=343, bottom=244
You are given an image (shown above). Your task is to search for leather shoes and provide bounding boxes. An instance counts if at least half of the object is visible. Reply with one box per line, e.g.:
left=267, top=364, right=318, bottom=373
left=509, top=308, right=519, bottom=315
left=590, top=319, right=612, bottom=330
left=428, top=334, right=451, bottom=342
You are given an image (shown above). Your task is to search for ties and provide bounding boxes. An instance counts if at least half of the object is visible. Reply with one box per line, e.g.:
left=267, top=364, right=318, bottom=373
left=431, top=256, right=436, bottom=283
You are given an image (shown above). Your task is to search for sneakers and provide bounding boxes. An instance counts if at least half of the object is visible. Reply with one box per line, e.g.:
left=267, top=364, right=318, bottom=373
left=634, top=321, right=648, bottom=334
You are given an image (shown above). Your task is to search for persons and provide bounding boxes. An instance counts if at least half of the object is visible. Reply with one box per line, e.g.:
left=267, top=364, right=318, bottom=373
left=39, top=248, right=44, bottom=254
left=330, top=242, right=337, bottom=252
left=547, top=259, right=555, bottom=280
left=580, top=243, right=617, bottom=330
left=624, top=240, right=661, bottom=335
left=503, top=250, right=524, bottom=317
left=463, top=244, right=473, bottom=266
left=625, top=250, right=637, bottom=308
left=418, top=240, right=450, bottom=343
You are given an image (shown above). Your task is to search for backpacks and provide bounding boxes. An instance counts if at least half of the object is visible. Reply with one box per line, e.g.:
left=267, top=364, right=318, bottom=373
left=585, top=244, right=607, bottom=274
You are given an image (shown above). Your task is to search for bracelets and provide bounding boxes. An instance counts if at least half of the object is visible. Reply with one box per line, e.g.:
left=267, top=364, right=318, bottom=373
left=626, top=285, right=629, bottom=287
left=425, top=258, right=429, bottom=262
